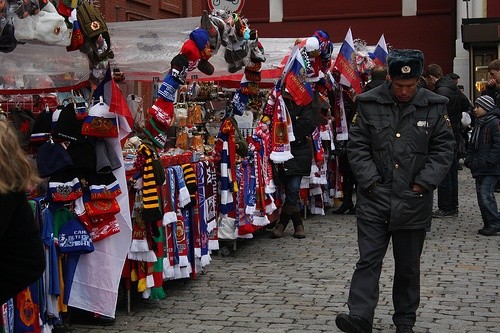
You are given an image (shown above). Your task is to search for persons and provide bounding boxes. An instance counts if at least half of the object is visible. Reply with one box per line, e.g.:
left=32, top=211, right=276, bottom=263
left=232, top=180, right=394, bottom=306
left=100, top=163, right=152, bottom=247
left=334, top=50, right=455, bottom=333
left=270, top=59, right=500, bottom=239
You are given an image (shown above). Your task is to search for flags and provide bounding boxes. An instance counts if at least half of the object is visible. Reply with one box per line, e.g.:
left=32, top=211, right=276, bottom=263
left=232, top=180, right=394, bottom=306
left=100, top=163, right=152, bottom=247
left=372, top=34, right=389, bottom=68
left=272, top=45, right=314, bottom=107
left=333, top=27, right=362, bottom=95
left=108, top=78, right=136, bottom=130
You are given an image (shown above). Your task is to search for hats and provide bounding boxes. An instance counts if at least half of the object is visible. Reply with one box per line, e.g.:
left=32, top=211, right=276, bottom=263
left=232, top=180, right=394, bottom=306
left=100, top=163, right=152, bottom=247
left=475, top=95, right=495, bottom=112
left=386, top=48, right=424, bottom=80
left=0, top=0, right=123, bottom=256
left=141, top=9, right=266, bottom=156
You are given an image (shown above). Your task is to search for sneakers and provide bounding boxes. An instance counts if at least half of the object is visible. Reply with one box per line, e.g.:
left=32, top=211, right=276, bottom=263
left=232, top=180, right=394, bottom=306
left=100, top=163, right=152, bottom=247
left=478, top=226, right=500, bottom=235
left=432, top=209, right=453, bottom=218
left=335, top=313, right=372, bottom=333
left=453, top=207, right=459, bottom=217
left=396, top=326, right=415, bottom=333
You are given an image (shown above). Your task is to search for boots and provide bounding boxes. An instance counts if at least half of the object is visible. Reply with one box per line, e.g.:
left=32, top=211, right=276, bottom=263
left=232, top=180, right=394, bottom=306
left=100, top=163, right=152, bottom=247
left=271, top=204, right=294, bottom=238
left=286, top=204, right=306, bottom=239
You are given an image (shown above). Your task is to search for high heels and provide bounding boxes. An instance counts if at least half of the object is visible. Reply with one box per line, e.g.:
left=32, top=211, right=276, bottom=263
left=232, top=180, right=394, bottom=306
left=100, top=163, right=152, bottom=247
left=332, top=201, right=354, bottom=215
left=347, top=206, right=355, bottom=215
